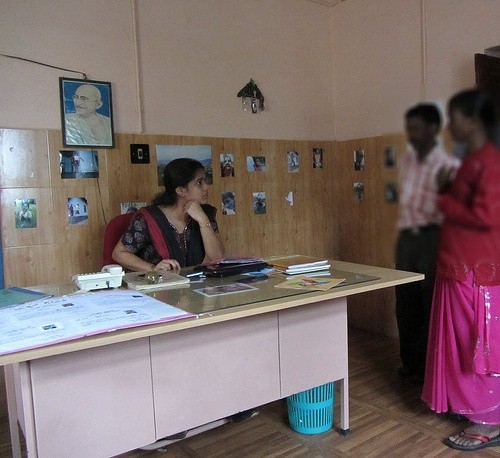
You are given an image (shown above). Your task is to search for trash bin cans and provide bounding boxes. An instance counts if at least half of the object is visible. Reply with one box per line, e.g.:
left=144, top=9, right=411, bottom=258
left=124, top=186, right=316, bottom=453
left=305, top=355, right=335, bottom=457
left=287, top=382, right=334, bottom=435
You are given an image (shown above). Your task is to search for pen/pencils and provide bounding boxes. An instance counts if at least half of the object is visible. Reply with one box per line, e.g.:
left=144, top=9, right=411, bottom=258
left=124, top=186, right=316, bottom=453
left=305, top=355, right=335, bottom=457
left=186, top=272, right=204, bottom=278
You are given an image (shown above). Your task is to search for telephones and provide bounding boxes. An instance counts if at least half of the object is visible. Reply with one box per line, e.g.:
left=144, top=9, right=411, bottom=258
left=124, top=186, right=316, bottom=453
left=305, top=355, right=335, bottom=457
left=71, top=265, right=125, bottom=291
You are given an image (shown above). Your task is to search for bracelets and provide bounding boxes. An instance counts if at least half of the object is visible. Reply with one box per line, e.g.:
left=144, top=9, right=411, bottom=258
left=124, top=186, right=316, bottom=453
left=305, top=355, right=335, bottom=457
left=150, top=264, right=156, bottom=272
left=200, top=220, right=211, bottom=229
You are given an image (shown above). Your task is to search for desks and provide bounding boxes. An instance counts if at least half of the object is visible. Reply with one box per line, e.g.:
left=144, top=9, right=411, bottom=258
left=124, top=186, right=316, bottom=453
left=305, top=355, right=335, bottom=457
left=0, top=255, right=425, bottom=458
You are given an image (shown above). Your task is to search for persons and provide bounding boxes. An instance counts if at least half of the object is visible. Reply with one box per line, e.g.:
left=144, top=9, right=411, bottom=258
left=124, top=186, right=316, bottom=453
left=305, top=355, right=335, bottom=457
left=72, top=152, right=84, bottom=177
left=68, top=203, right=80, bottom=220
left=253, top=157, right=263, bottom=172
left=65, top=84, right=112, bottom=146
left=221, top=155, right=233, bottom=178
left=314, top=149, right=321, bottom=164
left=290, top=152, right=296, bottom=166
left=391, top=100, right=462, bottom=384
left=20, top=203, right=32, bottom=227
left=385, top=148, right=394, bottom=167
left=109, top=157, right=262, bottom=441
left=421, top=87, right=500, bottom=450
left=354, top=150, right=364, bottom=171
left=222, top=192, right=235, bottom=215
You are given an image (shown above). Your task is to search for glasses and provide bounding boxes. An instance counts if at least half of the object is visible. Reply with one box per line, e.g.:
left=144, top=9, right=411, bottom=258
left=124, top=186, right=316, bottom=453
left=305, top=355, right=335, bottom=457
left=72, top=94, right=98, bottom=103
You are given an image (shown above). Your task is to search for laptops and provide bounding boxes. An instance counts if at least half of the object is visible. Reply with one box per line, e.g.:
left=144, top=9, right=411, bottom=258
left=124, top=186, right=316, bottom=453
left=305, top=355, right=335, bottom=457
left=193, top=262, right=267, bottom=277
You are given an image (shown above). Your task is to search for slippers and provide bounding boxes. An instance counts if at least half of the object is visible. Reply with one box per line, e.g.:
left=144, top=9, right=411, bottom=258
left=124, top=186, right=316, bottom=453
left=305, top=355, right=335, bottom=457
left=447, top=428, right=500, bottom=451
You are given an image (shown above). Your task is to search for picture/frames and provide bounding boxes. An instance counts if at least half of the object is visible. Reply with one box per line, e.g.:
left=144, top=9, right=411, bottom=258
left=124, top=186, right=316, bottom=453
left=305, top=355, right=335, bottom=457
left=59, top=77, right=115, bottom=149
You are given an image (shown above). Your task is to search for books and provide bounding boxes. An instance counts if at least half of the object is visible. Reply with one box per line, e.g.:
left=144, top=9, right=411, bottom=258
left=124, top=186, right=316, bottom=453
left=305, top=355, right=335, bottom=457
left=121, top=268, right=191, bottom=291
left=267, top=255, right=331, bottom=275
